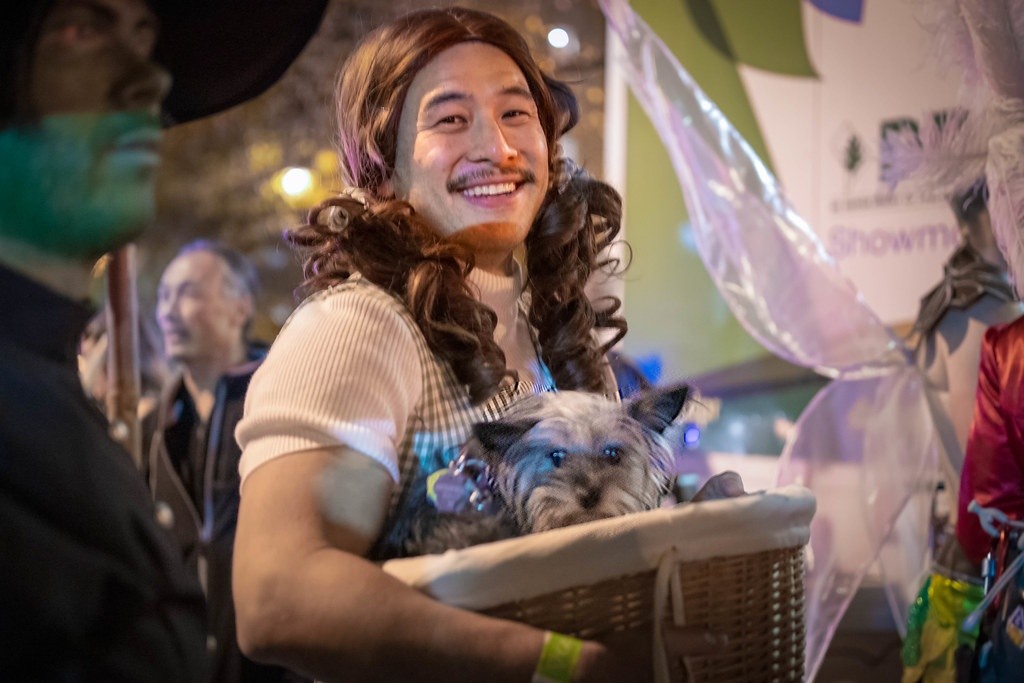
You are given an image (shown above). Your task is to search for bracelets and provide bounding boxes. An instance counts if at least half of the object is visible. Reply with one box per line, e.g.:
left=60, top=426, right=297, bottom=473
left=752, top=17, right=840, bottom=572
left=531, top=631, right=584, bottom=683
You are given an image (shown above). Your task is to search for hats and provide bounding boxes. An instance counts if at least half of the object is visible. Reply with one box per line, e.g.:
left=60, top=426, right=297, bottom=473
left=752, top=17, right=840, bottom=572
left=150, top=0, right=329, bottom=127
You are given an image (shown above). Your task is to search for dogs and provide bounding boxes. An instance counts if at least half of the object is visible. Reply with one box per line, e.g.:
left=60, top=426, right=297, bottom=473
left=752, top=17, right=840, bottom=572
left=402, top=382, right=691, bottom=554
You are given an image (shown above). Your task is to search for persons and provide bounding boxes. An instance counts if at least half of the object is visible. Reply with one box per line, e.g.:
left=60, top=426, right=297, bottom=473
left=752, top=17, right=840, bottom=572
left=0, top=0, right=628, bottom=683
left=951, top=308, right=1024, bottom=683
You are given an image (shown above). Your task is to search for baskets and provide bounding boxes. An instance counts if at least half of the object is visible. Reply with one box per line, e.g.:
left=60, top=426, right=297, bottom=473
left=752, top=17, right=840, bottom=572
left=370, top=485, right=817, bottom=683
